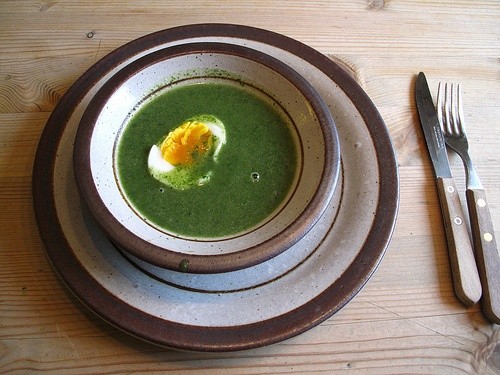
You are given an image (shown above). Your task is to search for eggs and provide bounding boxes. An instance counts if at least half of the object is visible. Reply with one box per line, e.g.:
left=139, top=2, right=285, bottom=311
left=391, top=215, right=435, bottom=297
left=147, top=114, right=226, bottom=191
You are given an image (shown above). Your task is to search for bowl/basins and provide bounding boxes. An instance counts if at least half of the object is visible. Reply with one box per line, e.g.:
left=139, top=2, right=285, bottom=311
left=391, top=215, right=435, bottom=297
left=72, top=41, right=340, bottom=275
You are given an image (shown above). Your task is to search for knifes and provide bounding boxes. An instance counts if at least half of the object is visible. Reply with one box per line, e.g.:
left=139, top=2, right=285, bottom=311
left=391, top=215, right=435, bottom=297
left=415, top=71, right=482, bottom=304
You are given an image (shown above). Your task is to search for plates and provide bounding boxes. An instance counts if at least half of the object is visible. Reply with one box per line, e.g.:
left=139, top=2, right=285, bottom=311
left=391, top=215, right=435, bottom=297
left=32, top=21, right=401, bottom=353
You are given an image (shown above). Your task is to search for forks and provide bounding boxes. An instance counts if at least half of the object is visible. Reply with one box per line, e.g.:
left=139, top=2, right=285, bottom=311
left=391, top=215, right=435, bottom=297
left=435, top=82, right=500, bottom=323
